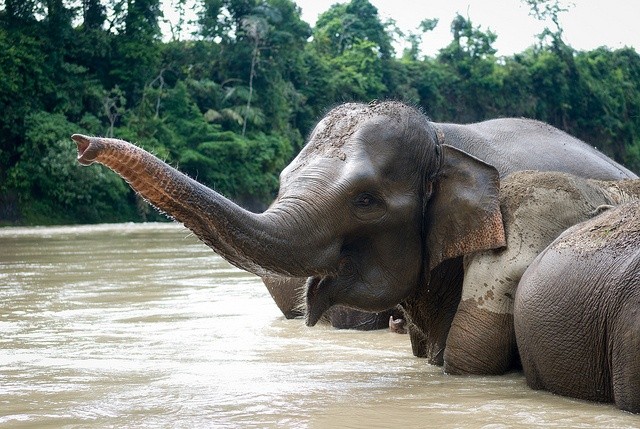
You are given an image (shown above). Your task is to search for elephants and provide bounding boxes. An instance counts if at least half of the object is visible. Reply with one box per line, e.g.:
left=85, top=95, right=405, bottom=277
left=71, top=97, right=640, bottom=366
left=513, top=196, right=640, bottom=416
left=443, top=169, right=640, bottom=378
